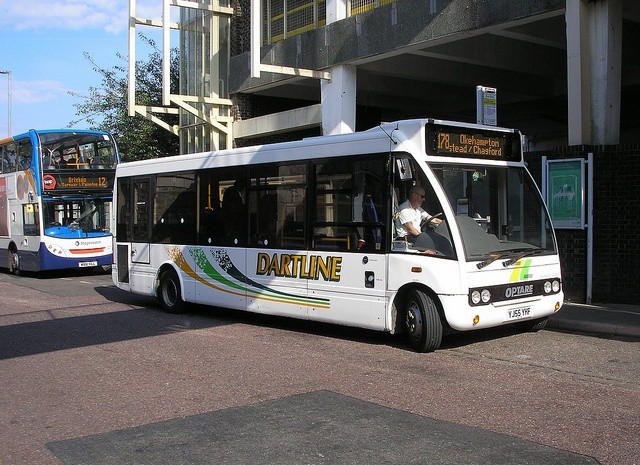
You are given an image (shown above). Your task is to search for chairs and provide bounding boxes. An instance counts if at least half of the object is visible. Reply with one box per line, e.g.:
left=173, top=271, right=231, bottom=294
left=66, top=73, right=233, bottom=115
left=90, top=156, right=108, bottom=169
left=78, top=156, right=90, bottom=169
left=43, top=156, right=55, bottom=169
left=25, top=226, right=36, bottom=235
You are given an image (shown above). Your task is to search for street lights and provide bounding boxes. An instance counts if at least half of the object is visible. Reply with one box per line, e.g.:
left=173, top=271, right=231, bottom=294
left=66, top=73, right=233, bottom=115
left=0, top=69, right=13, bottom=137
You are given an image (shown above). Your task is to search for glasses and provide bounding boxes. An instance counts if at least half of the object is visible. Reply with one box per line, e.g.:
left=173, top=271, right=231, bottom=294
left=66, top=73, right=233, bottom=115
left=414, top=192, right=425, bottom=198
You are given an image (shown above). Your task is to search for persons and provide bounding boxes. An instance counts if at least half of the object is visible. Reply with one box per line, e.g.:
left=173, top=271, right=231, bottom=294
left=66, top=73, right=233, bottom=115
left=337, top=170, right=376, bottom=251
left=394, top=184, right=444, bottom=237
left=221, top=177, right=251, bottom=207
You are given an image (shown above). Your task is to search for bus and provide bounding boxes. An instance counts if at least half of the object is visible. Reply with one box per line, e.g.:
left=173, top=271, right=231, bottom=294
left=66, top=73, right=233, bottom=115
left=111, top=119, right=564, bottom=352
left=0, top=128, right=122, bottom=277
